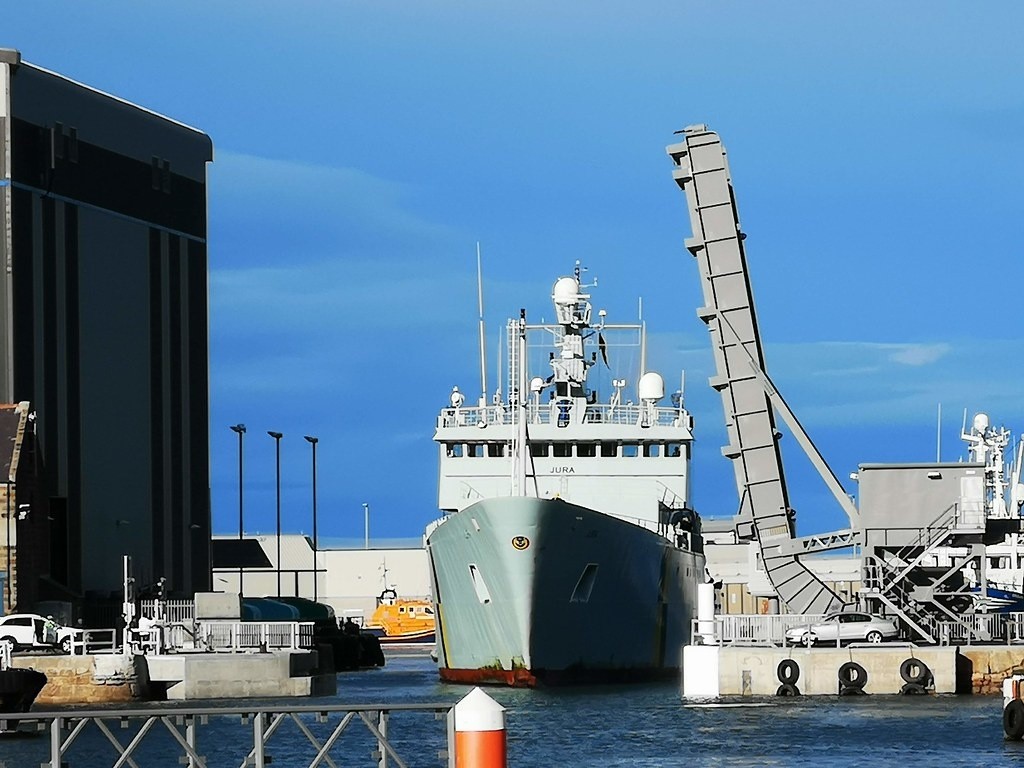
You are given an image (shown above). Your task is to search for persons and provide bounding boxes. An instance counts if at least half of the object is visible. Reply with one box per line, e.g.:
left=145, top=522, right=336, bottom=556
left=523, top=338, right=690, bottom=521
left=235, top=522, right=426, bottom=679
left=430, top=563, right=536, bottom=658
left=673, top=447, right=681, bottom=457
left=116, top=613, right=156, bottom=654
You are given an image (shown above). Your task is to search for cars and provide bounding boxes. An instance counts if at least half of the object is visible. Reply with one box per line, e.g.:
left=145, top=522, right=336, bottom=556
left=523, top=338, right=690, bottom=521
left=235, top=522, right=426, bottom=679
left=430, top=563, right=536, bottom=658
left=784, top=611, right=898, bottom=647
left=0, top=614, right=84, bottom=654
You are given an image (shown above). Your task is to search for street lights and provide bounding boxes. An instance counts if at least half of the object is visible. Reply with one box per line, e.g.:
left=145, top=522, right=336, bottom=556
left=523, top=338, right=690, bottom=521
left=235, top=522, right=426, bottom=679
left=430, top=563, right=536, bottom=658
left=266, top=430, right=282, bottom=597
left=229, top=424, right=244, bottom=598
left=303, top=436, right=318, bottom=603
left=361, top=503, right=370, bottom=549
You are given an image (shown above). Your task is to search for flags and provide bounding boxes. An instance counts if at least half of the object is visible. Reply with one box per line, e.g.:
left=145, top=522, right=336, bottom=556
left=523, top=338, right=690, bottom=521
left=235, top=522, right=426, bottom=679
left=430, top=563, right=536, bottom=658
left=598, top=334, right=608, bottom=365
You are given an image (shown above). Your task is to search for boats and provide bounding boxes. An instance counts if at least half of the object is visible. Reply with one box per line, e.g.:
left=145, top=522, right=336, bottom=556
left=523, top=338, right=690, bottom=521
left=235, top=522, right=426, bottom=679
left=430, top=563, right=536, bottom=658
left=922, top=401, right=1024, bottom=602
left=1002, top=669, right=1024, bottom=742
left=363, top=599, right=435, bottom=643
left=420, top=238, right=706, bottom=689
